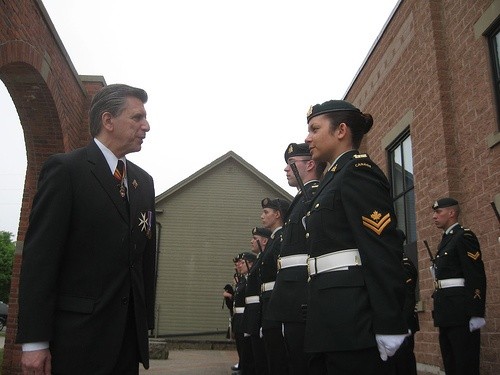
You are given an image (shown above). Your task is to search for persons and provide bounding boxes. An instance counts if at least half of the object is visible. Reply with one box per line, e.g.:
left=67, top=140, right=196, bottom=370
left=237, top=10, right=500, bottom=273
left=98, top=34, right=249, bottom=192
left=223, top=99, right=419, bottom=375
left=430, top=198, right=486, bottom=375
left=15, top=84, right=154, bottom=375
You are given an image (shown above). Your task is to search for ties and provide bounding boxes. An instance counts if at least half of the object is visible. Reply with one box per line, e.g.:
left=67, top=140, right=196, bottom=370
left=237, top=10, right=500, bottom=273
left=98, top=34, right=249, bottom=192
left=441, top=233, right=448, bottom=245
left=114, top=159, right=127, bottom=199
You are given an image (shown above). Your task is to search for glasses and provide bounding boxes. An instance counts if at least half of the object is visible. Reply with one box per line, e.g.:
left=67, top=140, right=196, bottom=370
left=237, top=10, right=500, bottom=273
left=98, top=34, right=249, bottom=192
left=288, top=159, right=310, bottom=169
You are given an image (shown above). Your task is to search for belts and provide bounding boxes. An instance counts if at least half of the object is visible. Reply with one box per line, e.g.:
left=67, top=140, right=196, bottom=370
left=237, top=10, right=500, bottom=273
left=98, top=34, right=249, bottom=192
left=433, top=278, right=465, bottom=288
left=277, top=254, right=310, bottom=270
left=260, top=281, right=275, bottom=292
left=234, top=307, right=245, bottom=313
left=308, top=249, right=362, bottom=275
left=244, top=296, right=260, bottom=304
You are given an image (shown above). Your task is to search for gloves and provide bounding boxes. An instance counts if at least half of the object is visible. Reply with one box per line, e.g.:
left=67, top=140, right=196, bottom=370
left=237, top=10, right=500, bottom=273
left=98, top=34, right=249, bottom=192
left=244, top=333, right=251, bottom=337
left=469, top=318, right=484, bottom=332
left=376, top=334, right=409, bottom=360
left=260, top=327, right=263, bottom=338
left=281, top=321, right=285, bottom=337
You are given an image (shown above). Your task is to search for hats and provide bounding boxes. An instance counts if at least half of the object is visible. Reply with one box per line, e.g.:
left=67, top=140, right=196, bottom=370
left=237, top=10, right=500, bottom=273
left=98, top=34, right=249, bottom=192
left=285, top=143, right=314, bottom=164
left=431, top=198, right=458, bottom=209
left=232, top=252, right=257, bottom=262
left=262, top=198, right=290, bottom=212
left=252, top=227, right=272, bottom=238
left=307, top=100, right=359, bottom=123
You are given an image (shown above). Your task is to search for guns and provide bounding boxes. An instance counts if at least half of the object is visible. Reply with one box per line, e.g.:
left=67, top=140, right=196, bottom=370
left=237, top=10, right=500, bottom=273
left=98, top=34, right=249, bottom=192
left=489, top=201, right=500, bottom=228
left=422, top=240, right=435, bottom=268
left=290, top=163, right=310, bottom=206
left=244, top=258, right=251, bottom=275
left=234, top=268, right=240, bottom=288
left=256, top=239, right=263, bottom=253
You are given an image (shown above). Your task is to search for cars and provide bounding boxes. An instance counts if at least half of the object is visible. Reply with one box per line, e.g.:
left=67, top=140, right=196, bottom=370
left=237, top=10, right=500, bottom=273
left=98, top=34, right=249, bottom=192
left=0, top=300, right=9, bottom=331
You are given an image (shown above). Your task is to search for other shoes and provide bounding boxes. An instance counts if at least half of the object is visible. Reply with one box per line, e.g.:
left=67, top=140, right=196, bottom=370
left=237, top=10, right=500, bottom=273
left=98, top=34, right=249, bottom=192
left=232, top=372, right=238, bottom=375
left=231, top=365, right=238, bottom=370
